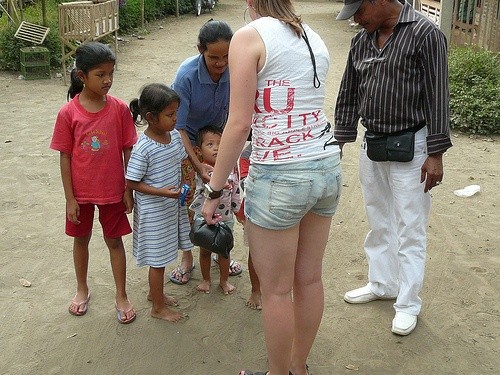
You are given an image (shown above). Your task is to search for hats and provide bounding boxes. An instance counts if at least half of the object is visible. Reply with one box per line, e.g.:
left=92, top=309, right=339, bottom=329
left=335, top=0, right=362, bottom=21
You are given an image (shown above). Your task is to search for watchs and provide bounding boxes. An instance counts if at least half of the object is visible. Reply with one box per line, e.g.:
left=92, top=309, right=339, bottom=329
left=202, top=181, right=225, bottom=199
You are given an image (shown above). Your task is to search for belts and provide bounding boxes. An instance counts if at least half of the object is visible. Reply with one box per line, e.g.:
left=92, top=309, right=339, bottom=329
left=367, top=123, right=427, bottom=136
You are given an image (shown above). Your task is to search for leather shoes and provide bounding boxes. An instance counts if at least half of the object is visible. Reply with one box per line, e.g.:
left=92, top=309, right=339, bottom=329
left=391, top=312, right=418, bottom=335
left=344, top=286, right=398, bottom=304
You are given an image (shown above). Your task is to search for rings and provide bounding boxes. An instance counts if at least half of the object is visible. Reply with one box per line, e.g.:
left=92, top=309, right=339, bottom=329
left=435, top=181, right=440, bottom=185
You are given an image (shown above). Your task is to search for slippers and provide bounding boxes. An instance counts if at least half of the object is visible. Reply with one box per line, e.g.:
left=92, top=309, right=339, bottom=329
left=238, top=369, right=269, bottom=375
left=169, top=262, right=194, bottom=284
left=214, top=255, right=242, bottom=275
left=69, top=291, right=91, bottom=316
left=114, top=299, right=135, bottom=322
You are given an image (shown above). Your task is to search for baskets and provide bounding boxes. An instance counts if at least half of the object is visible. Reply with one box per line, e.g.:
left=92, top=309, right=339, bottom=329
left=20, top=47, right=51, bottom=65
left=21, top=63, right=51, bottom=79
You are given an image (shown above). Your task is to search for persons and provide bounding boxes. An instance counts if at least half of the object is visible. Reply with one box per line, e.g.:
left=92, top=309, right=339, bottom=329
left=238, top=140, right=262, bottom=311
left=190, top=124, right=242, bottom=295
left=200, top=0, right=343, bottom=375
left=47, top=42, right=138, bottom=324
left=123, top=84, right=191, bottom=321
left=330, top=0, right=452, bottom=334
left=169, top=18, right=243, bottom=284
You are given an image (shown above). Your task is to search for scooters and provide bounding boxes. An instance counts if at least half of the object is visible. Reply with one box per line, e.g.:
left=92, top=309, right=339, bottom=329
left=195, top=0, right=215, bottom=16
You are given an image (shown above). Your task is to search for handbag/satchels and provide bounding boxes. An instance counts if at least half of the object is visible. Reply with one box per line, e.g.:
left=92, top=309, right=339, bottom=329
left=189, top=218, right=234, bottom=256
left=367, top=132, right=416, bottom=163
